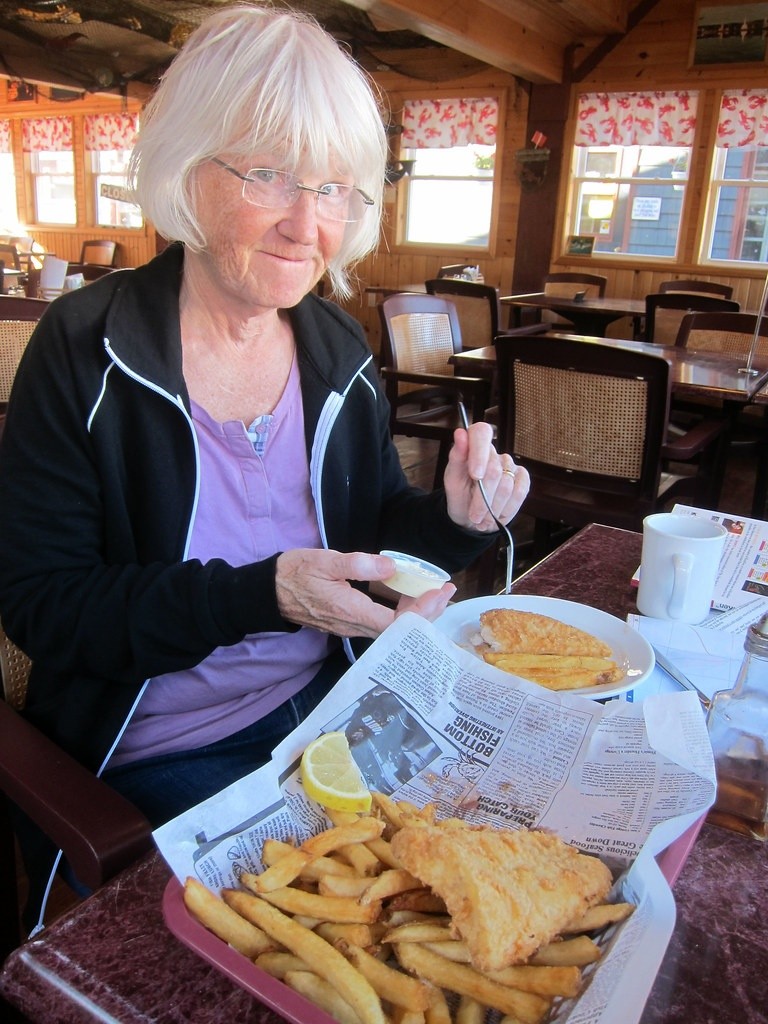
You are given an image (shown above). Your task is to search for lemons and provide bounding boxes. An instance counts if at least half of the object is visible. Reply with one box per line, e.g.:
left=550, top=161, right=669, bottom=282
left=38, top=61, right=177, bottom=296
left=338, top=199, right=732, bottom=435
left=299, top=733, right=372, bottom=814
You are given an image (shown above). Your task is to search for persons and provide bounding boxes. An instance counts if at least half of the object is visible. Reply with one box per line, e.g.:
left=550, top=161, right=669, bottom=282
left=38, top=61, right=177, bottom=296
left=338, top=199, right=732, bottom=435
left=0, top=1, right=530, bottom=831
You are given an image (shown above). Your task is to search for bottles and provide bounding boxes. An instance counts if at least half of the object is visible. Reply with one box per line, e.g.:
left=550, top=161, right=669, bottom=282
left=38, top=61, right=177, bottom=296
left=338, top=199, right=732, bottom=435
left=453, top=274, right=467, bottom=280
left=7, top=285, right=26, bottom=297
left=696, top=611, right=768, bottom=844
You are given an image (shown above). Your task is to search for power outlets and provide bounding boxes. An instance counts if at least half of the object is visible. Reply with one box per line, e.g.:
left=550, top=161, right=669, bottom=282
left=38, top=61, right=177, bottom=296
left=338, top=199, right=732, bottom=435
left=368, top=292, right=376, bottom=307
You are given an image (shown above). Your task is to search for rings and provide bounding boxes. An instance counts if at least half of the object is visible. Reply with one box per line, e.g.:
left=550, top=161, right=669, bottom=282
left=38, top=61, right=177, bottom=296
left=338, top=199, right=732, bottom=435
left=502, top=469, right=515, bottom=482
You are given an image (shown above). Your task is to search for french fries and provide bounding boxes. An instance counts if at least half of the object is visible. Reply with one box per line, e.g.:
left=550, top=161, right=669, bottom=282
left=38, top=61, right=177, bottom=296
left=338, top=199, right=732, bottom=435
left=485, top=649, right=623, bottom=689
left=183, top=790, right=633, bottom=1024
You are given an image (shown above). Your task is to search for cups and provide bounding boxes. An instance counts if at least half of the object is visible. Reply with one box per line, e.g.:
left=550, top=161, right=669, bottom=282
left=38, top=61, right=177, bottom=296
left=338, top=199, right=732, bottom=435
left=635, top=512, right=728, bottom=627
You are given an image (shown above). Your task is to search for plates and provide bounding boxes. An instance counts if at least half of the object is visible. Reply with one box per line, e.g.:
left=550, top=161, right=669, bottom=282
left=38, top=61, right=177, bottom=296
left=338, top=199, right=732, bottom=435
left=429, top=591, right=658, bottom=698
left=157, top=769, right=720, bottom=1024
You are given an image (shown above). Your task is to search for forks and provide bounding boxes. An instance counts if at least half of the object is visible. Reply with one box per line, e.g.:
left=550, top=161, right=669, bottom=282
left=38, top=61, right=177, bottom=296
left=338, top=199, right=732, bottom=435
left=456, top=400, right=515, bottom=595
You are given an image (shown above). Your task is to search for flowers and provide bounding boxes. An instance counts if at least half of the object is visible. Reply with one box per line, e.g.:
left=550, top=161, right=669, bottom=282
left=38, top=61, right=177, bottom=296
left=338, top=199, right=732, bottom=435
left=668, top=157, right=687, bottom=172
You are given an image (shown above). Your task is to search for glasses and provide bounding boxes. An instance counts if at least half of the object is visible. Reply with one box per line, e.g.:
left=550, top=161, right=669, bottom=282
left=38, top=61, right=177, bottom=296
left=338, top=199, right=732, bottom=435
left=211, top=155, right=374, bottom=224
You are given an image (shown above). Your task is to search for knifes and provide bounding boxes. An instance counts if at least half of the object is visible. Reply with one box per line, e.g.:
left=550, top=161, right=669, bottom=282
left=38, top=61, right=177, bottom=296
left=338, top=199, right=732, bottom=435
left=649, top=644, right=712, bottom=711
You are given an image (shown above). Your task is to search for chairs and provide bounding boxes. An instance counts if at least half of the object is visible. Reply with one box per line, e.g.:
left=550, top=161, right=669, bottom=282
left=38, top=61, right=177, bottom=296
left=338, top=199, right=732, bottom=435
left=436, top=264, right=476, bottom=279
left=424, top=279, right=553, bottom=353
left=0, top=295, right=499, bottom=1024
left=665, top=311, right=768, bottom=442
left=0, top=234, right=119, bottom=296
left=645, top=294, right=741, bottom=346
left=494, top=333, right=734, bottom=573
left=533, top=272, right=607, bottom=331
left=659, top=280, right=732, bottom=300
left=379, top=291, right=492, bottom=499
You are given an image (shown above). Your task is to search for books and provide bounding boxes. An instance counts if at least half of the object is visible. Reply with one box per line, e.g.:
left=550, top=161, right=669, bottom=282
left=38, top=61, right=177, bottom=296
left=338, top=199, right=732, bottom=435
left=629, top=502, right=767, bottom=612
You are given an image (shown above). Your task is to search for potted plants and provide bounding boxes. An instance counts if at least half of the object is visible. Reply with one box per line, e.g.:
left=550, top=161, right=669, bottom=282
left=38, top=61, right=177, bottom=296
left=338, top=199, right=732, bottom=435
left=473, top=151, right=494, bottom=185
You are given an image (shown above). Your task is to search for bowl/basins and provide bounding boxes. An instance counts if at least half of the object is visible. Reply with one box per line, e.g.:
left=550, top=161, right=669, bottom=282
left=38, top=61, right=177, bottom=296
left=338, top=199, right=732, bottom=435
left=378, top=551, right=451, bottom=599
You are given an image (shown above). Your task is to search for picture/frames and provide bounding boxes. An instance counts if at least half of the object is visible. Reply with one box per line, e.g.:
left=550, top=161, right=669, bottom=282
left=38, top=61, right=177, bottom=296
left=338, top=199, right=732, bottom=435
left=564, top=234, right=595, bottom=257
left=686, top=0, right=768, bottom=70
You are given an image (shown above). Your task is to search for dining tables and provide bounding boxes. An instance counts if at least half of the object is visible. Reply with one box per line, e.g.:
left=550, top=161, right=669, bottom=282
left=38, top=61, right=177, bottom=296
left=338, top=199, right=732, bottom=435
left=1, top=521, right=768, bottom=1024
left=447, top=331, right=767, bottom=522
left=498, top=292, right=647, bottom=339
left=364, top=284, right=429, bottom=294
